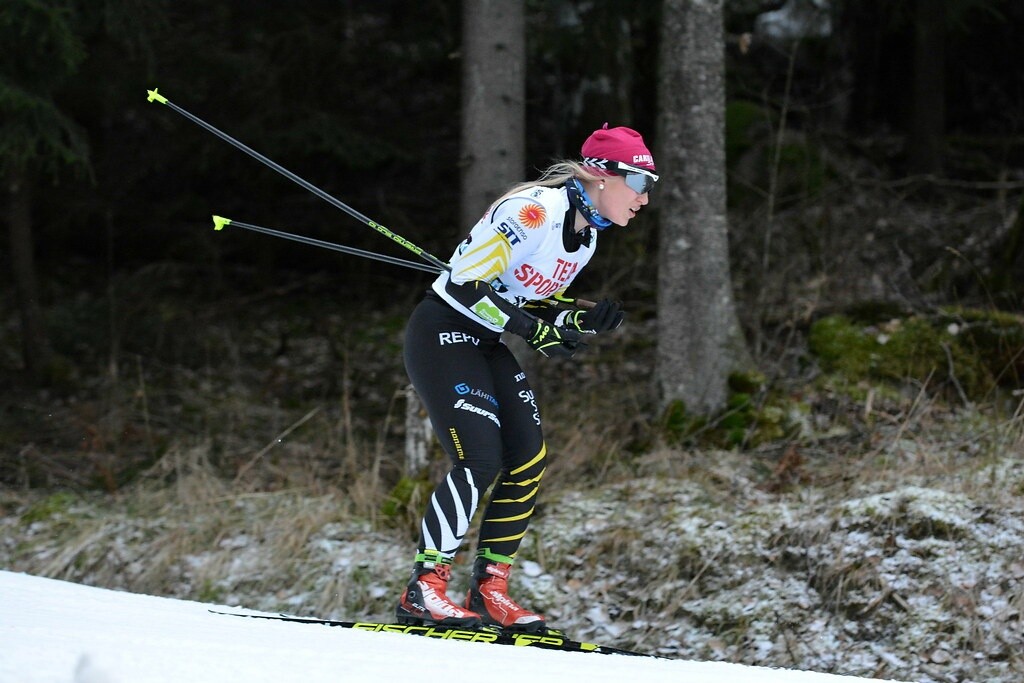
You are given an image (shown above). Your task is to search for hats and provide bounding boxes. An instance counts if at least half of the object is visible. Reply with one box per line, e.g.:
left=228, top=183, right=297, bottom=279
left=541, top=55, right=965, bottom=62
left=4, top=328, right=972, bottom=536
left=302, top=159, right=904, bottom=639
left=581, top=122, right=656, bottom=177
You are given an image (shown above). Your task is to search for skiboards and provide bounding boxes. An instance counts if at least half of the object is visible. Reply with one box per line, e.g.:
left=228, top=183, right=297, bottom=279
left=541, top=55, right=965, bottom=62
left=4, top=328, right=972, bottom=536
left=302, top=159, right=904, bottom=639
left=203, top=608, right=675, bottom=664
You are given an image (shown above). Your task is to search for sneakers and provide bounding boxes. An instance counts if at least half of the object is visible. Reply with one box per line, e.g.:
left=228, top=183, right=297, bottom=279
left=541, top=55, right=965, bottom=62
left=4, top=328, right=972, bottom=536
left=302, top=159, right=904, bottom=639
left=397, top=562, right=481, bottom=627
left=462, top=556, right=547, bottom=632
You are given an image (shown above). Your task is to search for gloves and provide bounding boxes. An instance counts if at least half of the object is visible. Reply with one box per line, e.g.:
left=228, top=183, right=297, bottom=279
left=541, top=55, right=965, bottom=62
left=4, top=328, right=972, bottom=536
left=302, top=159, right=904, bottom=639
left=526, top=323, right=587, bottom=359
left=563, top=298, right=626, bottom=334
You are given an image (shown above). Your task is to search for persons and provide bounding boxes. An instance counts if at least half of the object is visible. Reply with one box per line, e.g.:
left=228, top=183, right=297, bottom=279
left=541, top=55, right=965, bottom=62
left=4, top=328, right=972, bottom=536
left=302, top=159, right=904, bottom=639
left=397, top=127, right=659, bottom=633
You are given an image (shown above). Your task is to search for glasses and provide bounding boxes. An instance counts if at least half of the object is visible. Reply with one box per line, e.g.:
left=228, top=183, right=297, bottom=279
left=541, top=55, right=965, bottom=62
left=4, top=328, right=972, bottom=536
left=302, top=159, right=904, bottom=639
left=613, top=161, right=659, bottom=194
